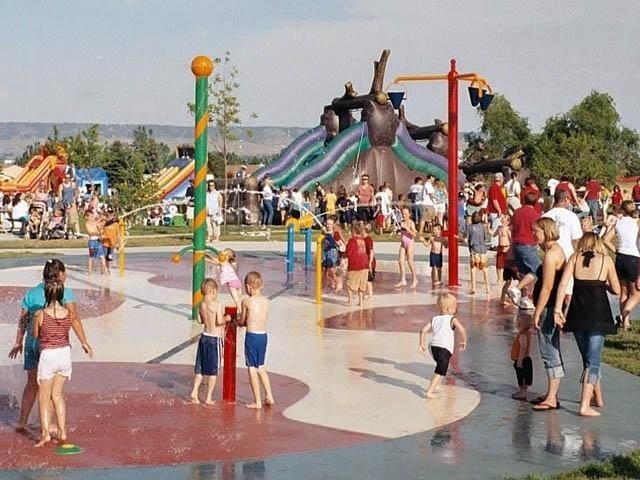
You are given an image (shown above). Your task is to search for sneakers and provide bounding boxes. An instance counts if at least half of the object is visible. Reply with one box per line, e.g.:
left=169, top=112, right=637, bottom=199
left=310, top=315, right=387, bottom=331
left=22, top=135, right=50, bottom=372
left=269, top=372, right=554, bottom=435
left=506, top=288, right=535, bottom=309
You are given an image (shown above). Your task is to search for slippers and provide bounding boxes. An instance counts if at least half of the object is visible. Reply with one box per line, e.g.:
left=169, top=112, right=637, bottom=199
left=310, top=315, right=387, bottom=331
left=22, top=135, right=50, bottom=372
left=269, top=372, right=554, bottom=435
left=530, top=397, right=560, bottom=411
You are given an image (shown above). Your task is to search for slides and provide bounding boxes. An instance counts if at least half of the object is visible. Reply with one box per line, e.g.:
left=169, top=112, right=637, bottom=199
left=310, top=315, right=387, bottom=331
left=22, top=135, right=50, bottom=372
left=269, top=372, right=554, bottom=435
left=1, top=156, right=55, bottom=193
left=251, top=120, right=466, bottom=197
left=142, top=158, right=196, bottom=200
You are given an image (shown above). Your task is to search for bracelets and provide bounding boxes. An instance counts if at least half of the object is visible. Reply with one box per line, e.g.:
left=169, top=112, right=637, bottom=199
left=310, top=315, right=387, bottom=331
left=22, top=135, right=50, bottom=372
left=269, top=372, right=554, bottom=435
left=553, top=308, right=563, bottom=313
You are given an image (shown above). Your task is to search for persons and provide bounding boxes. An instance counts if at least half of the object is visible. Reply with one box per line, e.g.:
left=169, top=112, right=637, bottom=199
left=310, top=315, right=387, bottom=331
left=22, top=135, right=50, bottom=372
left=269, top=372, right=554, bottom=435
left=187, top=278, right=231, bottom=406
left=458, top=172, right=640, bottom=335
left=553, top=231, right=622, bottom=417
left=413, top=294, right=467, bottom=399
left=31, top=278, right=75, bottom=449
left=532, top=215, right=568, bottom=410
left=235, top=272, right=275, bottom=409
left=7, top=258, right=93, bottom=433
left=185, top=179, right=195, bottom=228
left=84, top=190, right=118, bottom=275
left=206, top=181, right=224, bottom=243
left=2, top=172, right=80, bottom=239
left=259, top=173, right=450, bottom=306
left=508, top=313, right=534, bottom=400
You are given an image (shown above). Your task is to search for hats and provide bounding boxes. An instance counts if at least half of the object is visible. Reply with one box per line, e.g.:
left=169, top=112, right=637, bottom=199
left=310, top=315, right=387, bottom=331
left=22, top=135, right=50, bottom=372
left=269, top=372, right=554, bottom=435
left=494, top=172, right=504, bottom=181
